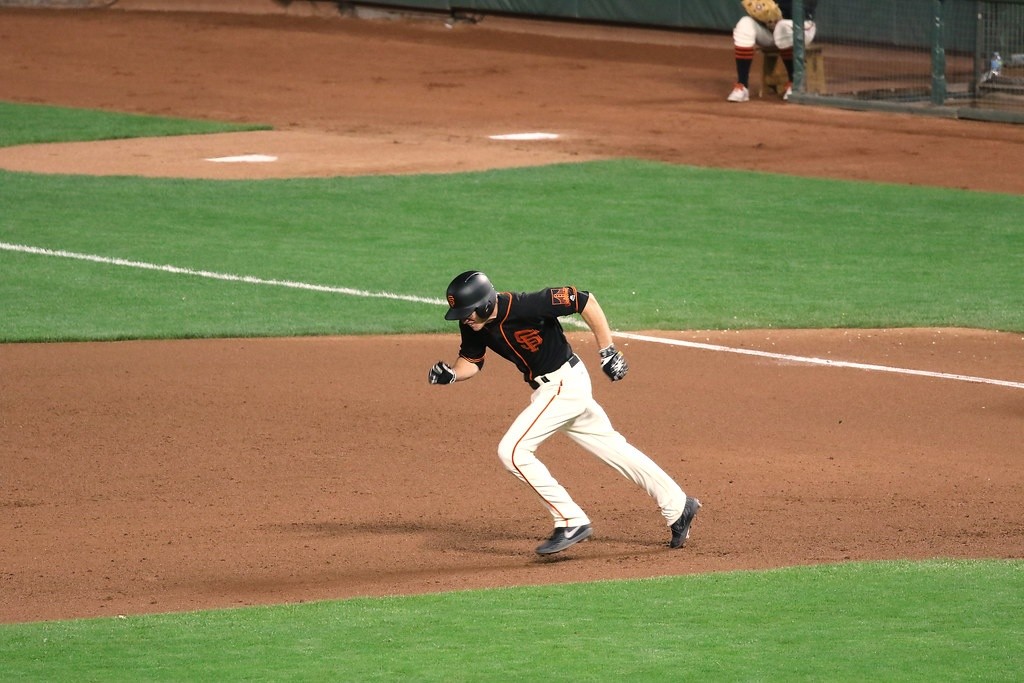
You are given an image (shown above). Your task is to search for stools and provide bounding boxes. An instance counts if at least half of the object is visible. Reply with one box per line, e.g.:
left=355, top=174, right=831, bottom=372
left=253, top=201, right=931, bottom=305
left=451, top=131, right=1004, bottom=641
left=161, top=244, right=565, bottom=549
left=756, top=45, right=826, bottom=96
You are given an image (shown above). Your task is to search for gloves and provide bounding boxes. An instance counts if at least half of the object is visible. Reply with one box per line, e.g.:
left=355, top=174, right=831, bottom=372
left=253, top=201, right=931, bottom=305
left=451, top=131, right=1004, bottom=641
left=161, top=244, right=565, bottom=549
left=428, top=362, right=456, bottom=385
left=599, top=343, right=628, bottom=381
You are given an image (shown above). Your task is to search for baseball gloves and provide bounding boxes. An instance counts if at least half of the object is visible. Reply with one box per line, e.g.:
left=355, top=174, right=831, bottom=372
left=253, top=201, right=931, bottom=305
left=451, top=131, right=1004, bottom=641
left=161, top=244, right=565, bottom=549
left=741, top=1, right=785, bottom=32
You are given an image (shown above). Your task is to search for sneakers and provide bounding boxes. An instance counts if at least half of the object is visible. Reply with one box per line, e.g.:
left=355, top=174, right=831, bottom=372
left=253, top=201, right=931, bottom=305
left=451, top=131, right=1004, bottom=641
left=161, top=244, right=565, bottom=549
left=783, top=81, right=792, bottom=99
left=670, top=496, right=702, bottom=548
left=728, top=83, right=749, bottom=102
left=536, top=524, right=593, bottom=554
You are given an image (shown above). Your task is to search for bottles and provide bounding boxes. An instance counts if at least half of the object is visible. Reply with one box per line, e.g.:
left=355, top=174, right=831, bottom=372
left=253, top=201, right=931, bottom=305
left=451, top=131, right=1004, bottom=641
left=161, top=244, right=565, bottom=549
left=991, top=51, right=1002, bottom=77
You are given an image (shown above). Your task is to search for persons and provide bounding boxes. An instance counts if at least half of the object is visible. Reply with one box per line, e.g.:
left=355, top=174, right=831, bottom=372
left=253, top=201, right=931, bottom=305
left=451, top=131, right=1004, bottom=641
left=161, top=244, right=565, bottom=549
left=427, top=271, right=701, bottom=558
left=727, top=0, right=818, bottom=102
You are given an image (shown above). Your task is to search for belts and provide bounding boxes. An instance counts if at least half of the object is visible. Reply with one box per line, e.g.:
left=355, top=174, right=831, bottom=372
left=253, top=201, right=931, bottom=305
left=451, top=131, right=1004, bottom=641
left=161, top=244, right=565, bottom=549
left=529, top=355, right=579, bottom=389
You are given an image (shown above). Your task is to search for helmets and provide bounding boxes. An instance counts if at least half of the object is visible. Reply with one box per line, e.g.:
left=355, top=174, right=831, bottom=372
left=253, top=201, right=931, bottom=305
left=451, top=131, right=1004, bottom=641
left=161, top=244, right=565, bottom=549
left=445, top=271, right=496, bottom=320
